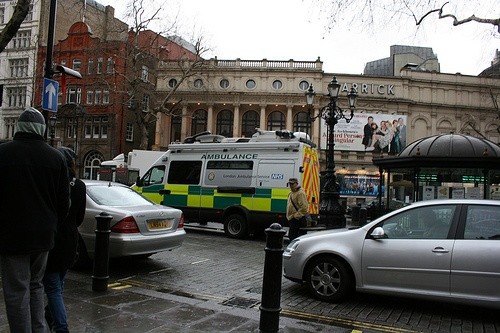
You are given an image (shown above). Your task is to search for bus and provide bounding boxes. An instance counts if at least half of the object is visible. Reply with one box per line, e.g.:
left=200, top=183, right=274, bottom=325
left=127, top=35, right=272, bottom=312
left=97, top=149, right=167, bottom=188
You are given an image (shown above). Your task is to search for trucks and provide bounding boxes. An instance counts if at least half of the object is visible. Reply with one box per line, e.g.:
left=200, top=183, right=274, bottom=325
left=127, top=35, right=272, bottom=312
left=129, top=128, right=321, bottom=239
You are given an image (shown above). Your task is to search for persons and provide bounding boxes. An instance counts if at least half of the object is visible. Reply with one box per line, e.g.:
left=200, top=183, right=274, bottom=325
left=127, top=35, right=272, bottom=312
left=0, top=106, right=86, bottom=333
left=286, top=178, right=308, bottom=242
left=363, top=116, right=406, bottom=155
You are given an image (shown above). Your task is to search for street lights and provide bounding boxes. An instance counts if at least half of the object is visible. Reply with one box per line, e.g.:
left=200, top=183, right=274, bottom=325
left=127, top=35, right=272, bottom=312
left=304, top=75, right=359, bottom=229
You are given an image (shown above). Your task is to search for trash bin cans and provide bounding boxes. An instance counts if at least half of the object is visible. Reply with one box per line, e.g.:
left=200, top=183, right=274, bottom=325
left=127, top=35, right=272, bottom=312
left=360, top=208, right=367, bottom=226
left=352, top=207, right=361, bottom=225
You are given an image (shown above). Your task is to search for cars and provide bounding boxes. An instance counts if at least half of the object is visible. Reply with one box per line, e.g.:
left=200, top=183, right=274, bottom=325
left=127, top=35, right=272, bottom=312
left=58, top=179, right=187, bottom=263
left=282, top=199, right=500, bottom=310
left=346, top=198, right=408, bottom=221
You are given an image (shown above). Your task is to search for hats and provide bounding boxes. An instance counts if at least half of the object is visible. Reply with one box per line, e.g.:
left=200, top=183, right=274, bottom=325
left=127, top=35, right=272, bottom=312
left=287, top=178, right=298, bottom=184
left=58, top=145, right=76, bottom=168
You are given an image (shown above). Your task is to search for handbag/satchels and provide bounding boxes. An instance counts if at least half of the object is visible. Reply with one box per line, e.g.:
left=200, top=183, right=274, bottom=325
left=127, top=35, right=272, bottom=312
left=302, top=214, right=312, bottom=224
left=62, top=218, right=79, bottom=273
